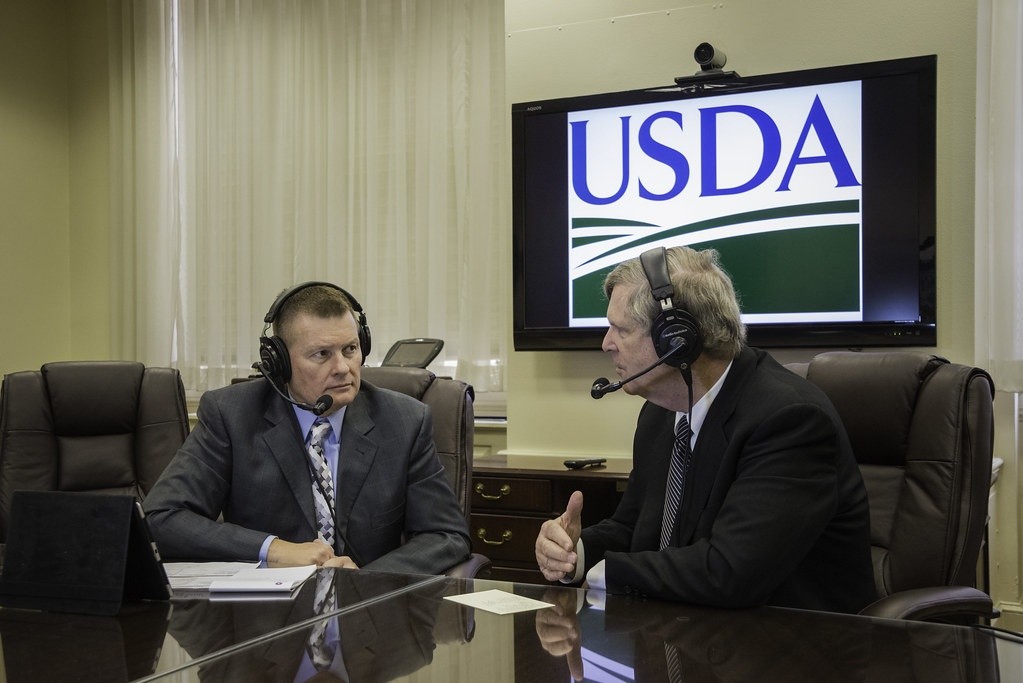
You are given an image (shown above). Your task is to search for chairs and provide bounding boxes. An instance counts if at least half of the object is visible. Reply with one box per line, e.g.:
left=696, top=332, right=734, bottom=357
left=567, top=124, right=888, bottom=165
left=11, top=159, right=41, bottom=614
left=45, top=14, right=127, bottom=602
left=230, top=369, right=493, bottom=580
left=807, top=350, right=1000, bottom=627
left=0, top=360, right=190, bottom=545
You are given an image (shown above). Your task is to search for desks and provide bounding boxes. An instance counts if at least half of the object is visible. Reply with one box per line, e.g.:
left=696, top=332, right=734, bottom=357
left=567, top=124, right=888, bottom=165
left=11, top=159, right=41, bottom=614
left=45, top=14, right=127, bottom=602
left=0, top=573, right=1023, bottom=683
left=474, top=453, right=633, bottom=586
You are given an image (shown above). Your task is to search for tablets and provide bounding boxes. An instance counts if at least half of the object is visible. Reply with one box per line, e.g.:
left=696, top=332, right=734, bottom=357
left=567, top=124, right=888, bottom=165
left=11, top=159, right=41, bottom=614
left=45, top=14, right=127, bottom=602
left=0, top=490, right=179, bottom=617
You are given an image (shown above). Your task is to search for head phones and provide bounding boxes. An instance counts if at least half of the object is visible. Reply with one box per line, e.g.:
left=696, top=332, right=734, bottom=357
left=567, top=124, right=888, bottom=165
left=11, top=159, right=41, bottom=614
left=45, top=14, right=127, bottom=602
left=258, top=280, right=371, bottom=385
left=640, top=246, right=704, bottom=368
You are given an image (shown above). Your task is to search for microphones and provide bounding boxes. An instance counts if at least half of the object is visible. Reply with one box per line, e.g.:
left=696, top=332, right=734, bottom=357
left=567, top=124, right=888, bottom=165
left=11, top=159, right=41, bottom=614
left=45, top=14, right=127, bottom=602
left=591, top=336, right=687, bottom=399
left=258, top=360, right=333, bottom=416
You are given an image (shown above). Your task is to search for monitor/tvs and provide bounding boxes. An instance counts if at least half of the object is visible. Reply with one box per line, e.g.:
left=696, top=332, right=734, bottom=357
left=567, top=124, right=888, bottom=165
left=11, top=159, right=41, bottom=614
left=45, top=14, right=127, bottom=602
left=381, top=338, right=444, bottom=369
left=512, top=55, right=938, bottom=350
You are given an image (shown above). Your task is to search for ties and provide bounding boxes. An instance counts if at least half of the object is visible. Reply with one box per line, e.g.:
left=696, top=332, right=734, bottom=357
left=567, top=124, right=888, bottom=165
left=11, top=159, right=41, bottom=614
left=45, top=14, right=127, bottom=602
left=663, top=643, right=687, bottom=682
left=307, top=421, right=334, bottom=550
left=661, top=416, right=696, bottom=551
left=307, top=567, right=339, bottom=670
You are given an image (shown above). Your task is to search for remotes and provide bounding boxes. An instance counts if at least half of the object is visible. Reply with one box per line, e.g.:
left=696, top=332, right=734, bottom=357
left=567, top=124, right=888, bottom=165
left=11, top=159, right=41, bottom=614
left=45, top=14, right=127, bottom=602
left=564, top=459, right=607, bottom=468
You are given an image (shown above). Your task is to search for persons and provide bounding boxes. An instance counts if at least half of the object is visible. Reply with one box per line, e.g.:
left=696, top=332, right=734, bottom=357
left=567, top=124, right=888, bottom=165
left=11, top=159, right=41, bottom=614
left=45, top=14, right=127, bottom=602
left=533, top=243, right=879, bottom=613
left=166, top=568, right=447, bottom=683
left=533, top=583, right=839, bottom=683
left=141, top=280, right=474, bottom=578
